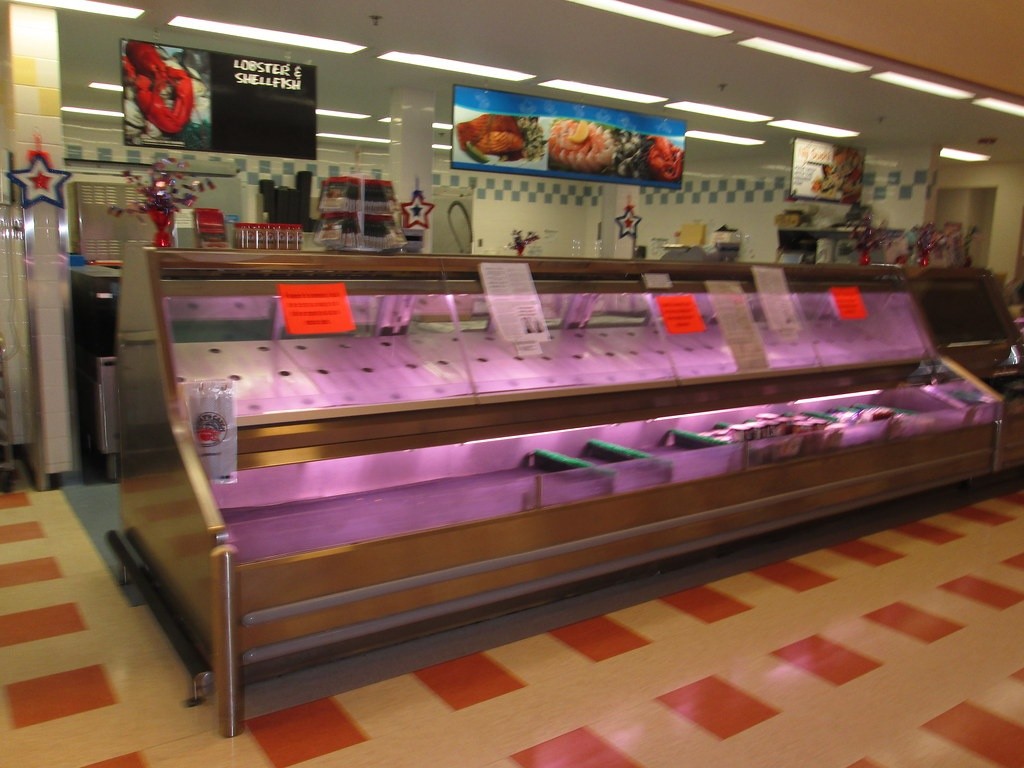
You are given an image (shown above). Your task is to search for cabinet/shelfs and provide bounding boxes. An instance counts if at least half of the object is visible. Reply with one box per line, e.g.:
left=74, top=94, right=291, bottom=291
left=104, top=236, right=1006, bottom=744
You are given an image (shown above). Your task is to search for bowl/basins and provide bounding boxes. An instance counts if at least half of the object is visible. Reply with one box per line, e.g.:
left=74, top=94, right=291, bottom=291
left=240, top=166, right=313, bottom=233
